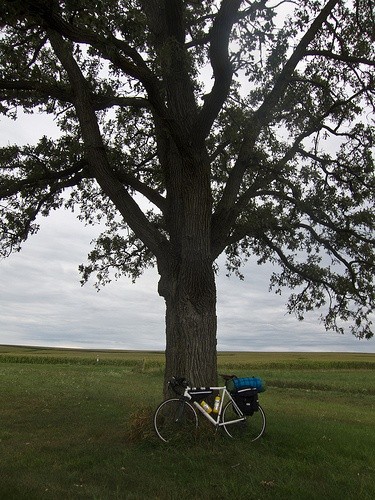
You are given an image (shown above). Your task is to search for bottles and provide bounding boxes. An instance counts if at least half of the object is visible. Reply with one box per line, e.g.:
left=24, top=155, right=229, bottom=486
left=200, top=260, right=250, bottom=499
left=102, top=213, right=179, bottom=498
left=199, top=400, right=212, bottom=414
left=212, top=393, right=221, bottom=413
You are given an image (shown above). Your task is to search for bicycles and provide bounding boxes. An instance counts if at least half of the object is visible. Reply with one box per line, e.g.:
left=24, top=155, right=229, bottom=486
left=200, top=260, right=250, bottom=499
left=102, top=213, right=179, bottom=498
left=153, top=374, right=266, bottom=445
